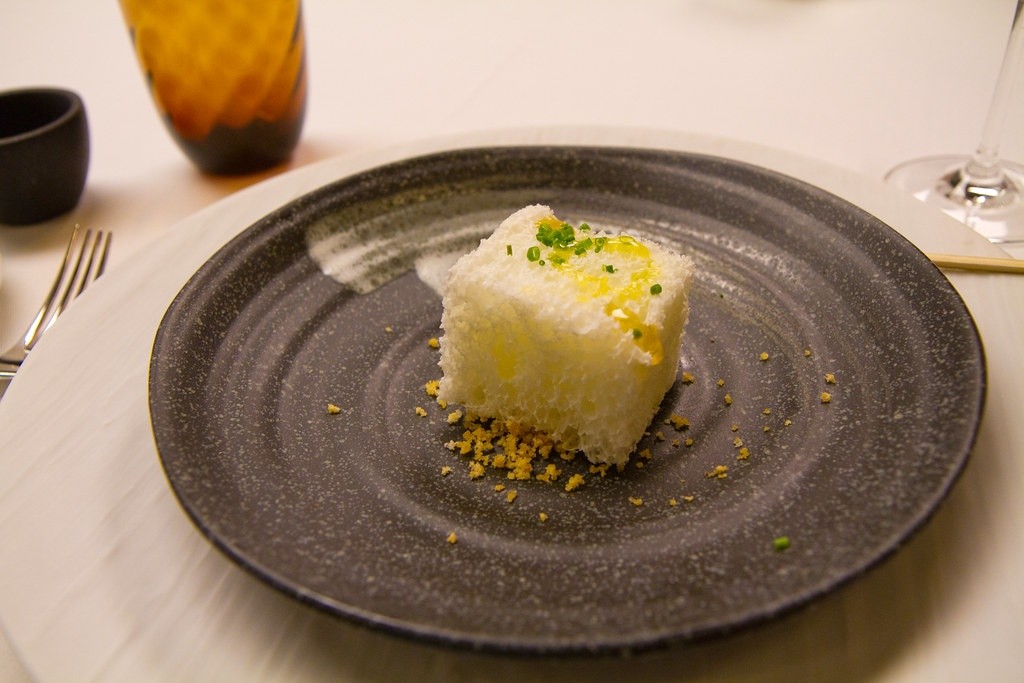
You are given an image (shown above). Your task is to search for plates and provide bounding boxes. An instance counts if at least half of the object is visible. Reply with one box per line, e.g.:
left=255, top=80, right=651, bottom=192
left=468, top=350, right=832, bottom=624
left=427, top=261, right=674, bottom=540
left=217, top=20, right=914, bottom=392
left=147, top=144, right=988, bottom=656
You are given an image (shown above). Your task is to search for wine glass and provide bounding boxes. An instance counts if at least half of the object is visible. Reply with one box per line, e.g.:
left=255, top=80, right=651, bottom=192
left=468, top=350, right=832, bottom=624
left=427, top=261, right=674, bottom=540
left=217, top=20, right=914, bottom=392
left=882, top=0, right=1024, bottom=246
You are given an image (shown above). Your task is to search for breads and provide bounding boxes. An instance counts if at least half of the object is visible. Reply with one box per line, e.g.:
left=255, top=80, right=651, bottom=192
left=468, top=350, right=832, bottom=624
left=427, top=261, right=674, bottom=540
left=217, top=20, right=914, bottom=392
left=425, top=201, right=700, bottom=477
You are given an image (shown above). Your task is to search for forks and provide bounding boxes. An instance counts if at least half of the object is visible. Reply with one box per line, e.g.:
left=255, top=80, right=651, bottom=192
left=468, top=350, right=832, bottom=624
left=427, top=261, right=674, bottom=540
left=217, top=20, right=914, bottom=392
left=0, top=223, right=113, bottom=380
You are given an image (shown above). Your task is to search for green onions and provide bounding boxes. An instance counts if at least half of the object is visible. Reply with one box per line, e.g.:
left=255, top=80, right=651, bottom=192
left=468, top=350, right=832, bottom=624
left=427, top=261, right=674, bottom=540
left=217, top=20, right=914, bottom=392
left=507, top=222, right=661, bottom=294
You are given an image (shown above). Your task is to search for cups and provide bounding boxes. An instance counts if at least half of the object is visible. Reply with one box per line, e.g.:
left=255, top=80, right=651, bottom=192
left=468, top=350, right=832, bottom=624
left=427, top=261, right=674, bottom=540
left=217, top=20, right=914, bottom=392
left=117, top=0, right=309, bottom=179
left=0, top=87, right=89, bottom=227
